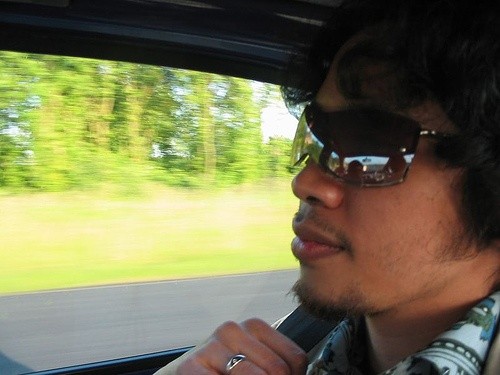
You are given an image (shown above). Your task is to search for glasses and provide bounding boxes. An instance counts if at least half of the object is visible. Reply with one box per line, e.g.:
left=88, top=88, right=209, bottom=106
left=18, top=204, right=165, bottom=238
left=290, top=101, right=462, bottom=188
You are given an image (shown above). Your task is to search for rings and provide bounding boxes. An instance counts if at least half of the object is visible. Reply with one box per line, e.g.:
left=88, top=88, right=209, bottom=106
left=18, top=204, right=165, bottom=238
left=225, top=354, right=246, bottom=374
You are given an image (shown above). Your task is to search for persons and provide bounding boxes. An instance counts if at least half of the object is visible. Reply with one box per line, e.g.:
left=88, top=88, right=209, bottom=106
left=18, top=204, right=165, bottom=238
left=146, top=0, right=500, bottom=375
